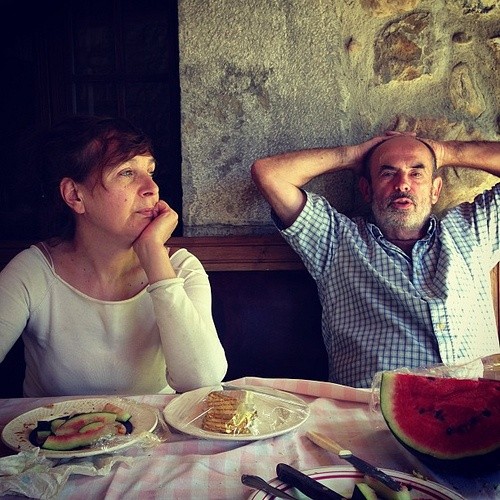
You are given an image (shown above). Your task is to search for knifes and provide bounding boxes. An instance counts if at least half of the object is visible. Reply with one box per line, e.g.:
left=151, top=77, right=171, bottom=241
left=306, top=428, right=402, bottom=491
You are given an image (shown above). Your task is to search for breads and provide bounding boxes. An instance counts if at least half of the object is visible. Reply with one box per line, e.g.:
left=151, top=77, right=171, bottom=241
left=202, top=390, right=257, bottom=434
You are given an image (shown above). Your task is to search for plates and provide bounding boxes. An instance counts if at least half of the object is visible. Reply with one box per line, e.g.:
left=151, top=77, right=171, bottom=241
left=1, top=395, right=159, bottom=461
left=162, top=384, right=311, bottom=442
left=245, top=464, right=466, bottom=500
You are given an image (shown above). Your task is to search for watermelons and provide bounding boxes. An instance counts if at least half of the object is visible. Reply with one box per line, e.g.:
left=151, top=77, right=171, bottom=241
left=36, top=403, right=134, bottom=450
left=378, top=371, right=500, bottom=464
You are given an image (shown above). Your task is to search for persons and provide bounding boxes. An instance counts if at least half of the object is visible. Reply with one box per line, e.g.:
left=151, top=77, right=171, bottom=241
left=0, top=119, right=234, bottom=399
left=249, top=128, right=500, bottom=395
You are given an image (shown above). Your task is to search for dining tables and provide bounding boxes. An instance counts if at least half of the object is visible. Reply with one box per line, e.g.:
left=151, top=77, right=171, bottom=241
left=1, top=377, right=500, bottom=500
